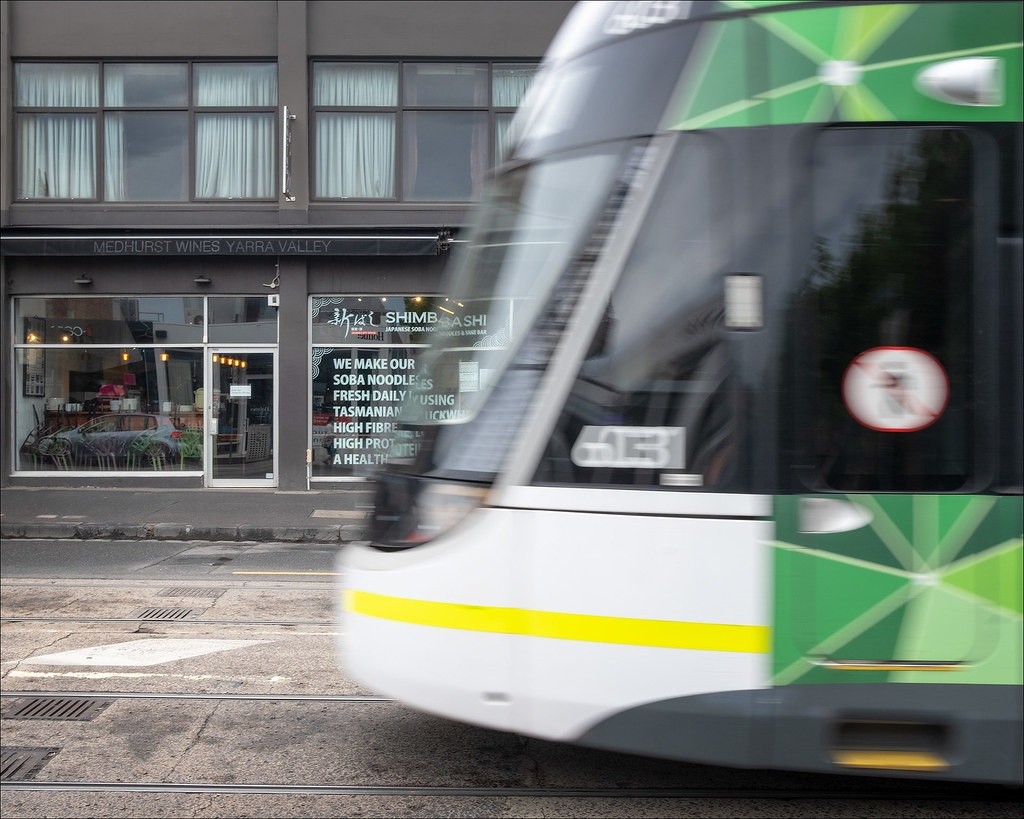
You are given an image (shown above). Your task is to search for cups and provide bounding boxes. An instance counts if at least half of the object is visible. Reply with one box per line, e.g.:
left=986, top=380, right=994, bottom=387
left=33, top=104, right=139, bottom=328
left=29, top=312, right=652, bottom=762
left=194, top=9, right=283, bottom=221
left=77, top=404, right=82, bottom=412
left=71, top=404, right=77, bottom=411
left=65, top=404, right=71, bottom=412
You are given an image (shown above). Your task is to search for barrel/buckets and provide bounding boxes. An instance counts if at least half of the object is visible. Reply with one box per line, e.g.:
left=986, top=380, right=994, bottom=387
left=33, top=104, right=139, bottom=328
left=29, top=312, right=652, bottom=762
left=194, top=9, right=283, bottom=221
left=195, top=388, right=221, bottom=411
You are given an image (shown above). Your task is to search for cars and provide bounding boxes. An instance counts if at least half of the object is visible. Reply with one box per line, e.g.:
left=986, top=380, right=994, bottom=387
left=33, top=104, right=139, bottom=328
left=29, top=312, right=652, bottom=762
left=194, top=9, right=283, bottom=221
left=36, top=411, right=183, bottom=469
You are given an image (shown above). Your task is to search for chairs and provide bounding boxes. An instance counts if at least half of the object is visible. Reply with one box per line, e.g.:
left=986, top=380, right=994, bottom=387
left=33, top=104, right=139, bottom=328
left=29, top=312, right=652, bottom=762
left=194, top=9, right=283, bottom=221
left=20, top=404, right=204, bottom=470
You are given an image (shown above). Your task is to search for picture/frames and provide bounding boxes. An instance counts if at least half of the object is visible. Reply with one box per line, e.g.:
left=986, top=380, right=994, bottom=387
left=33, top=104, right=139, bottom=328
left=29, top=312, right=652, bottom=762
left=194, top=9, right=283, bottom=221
left=23, top=316, right=46, bottom=398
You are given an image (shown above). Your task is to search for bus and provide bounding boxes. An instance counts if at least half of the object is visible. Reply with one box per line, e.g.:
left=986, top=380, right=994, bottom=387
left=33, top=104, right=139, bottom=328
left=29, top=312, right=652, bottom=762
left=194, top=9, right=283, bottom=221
left=330, top=0, right=1024, bottom=793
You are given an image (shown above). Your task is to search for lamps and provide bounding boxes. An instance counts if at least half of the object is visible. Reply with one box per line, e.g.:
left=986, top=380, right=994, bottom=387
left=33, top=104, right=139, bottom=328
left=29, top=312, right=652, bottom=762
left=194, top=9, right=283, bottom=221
left=213, top=353, right=247, bottom=368
left=73, top=274, right=92, bottom=283
left=121, top=349, right=128, bottom=360
left=193, top=275, right=211, bottom=282
left=160, top=348, right=169, bottom=361
